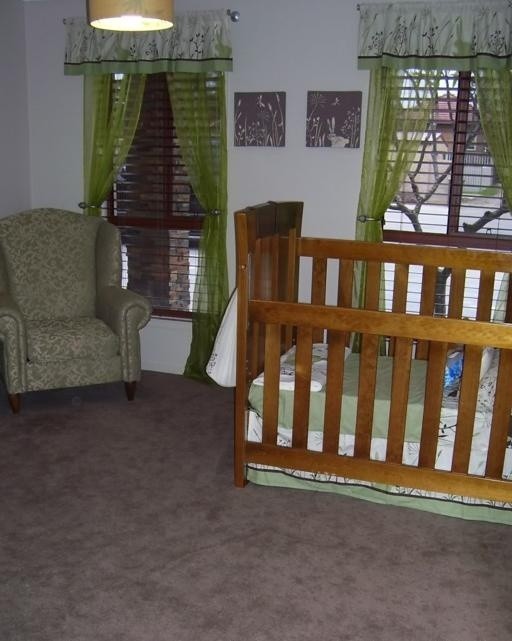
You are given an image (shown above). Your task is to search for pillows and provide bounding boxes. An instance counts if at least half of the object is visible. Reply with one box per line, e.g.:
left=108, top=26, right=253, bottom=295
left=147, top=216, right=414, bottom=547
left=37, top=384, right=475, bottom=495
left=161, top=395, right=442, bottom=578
left=479, top=347, right=494, bottom=382
left=441, top=347, right=464, bottom=407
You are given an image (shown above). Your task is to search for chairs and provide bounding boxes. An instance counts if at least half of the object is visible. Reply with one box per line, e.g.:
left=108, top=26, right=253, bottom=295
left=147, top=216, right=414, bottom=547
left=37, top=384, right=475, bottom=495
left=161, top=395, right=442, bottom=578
left=1, top=208, right=153, bottom=414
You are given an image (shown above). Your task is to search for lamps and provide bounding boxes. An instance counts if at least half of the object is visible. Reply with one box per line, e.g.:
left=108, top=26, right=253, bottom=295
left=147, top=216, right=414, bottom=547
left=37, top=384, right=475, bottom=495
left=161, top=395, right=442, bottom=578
left=88, top=0, right=174, bottom=32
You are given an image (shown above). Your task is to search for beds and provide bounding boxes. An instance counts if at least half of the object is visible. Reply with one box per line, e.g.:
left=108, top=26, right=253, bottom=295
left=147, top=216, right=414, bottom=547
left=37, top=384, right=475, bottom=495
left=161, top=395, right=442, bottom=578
left=234, top=200, right=512, bottom=526
